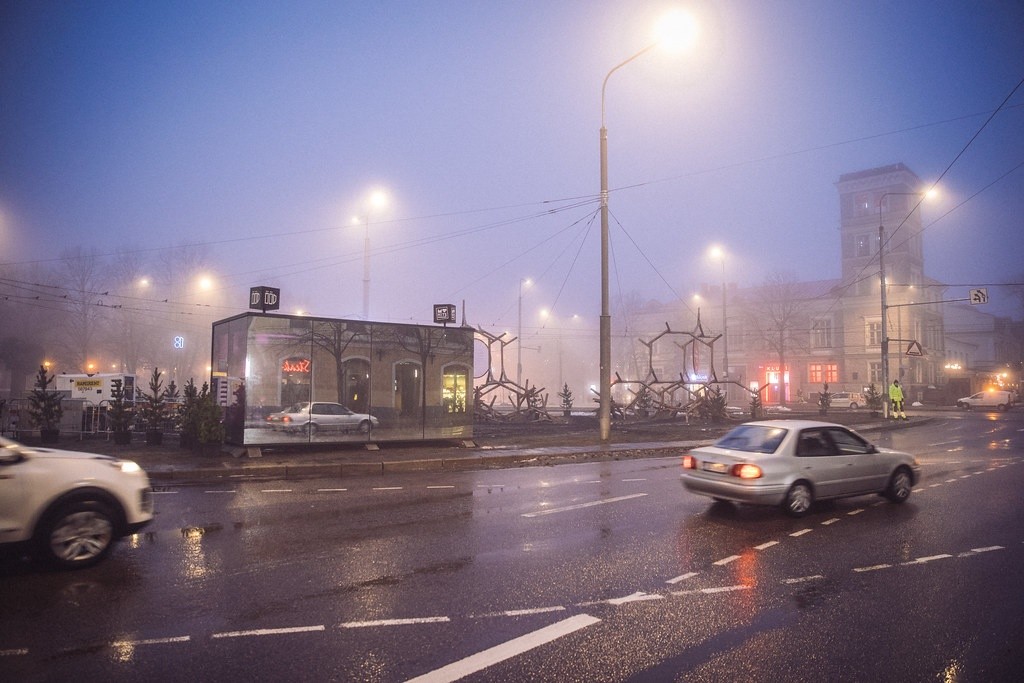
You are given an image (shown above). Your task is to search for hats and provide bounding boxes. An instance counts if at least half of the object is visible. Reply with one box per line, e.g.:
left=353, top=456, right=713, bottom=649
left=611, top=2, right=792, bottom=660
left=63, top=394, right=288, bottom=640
left=894, top=380, right=898, bottom=383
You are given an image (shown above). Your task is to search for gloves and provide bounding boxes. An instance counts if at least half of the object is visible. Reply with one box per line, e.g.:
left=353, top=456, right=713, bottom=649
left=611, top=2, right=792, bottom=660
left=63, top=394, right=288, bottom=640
left=892, top=399, right=894, bottom=403
left=902, top=398, right=904, bottom=401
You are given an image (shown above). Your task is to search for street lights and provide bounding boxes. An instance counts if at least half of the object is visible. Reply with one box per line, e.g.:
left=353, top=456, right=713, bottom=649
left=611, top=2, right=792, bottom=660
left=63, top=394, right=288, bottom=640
left=599, top=12, right=700, bottom=441
left=878, top=190, right=944, bottom=421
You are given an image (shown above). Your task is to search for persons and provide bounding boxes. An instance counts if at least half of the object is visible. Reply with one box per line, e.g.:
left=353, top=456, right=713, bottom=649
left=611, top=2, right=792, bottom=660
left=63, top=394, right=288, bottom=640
left=889, top=379, right=906, bottom=419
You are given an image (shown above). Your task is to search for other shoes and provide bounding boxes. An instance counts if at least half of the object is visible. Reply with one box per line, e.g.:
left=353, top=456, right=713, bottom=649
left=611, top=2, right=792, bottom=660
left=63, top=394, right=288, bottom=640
left=894, top=418, right=899, bottom=421
left=903, top=418, right=909, bottom=421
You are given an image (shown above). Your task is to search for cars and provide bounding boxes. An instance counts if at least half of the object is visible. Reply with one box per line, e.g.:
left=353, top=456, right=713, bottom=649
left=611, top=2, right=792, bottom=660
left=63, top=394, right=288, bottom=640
left=957, top=391, right=1014, bottom=412
left=819, top=392, right=868, bottom=410
left=266, top=402, right=379, bottom=437
left=678, top=419, right=922, bottom=518
left=0, top=435, right=155, bottom=572
